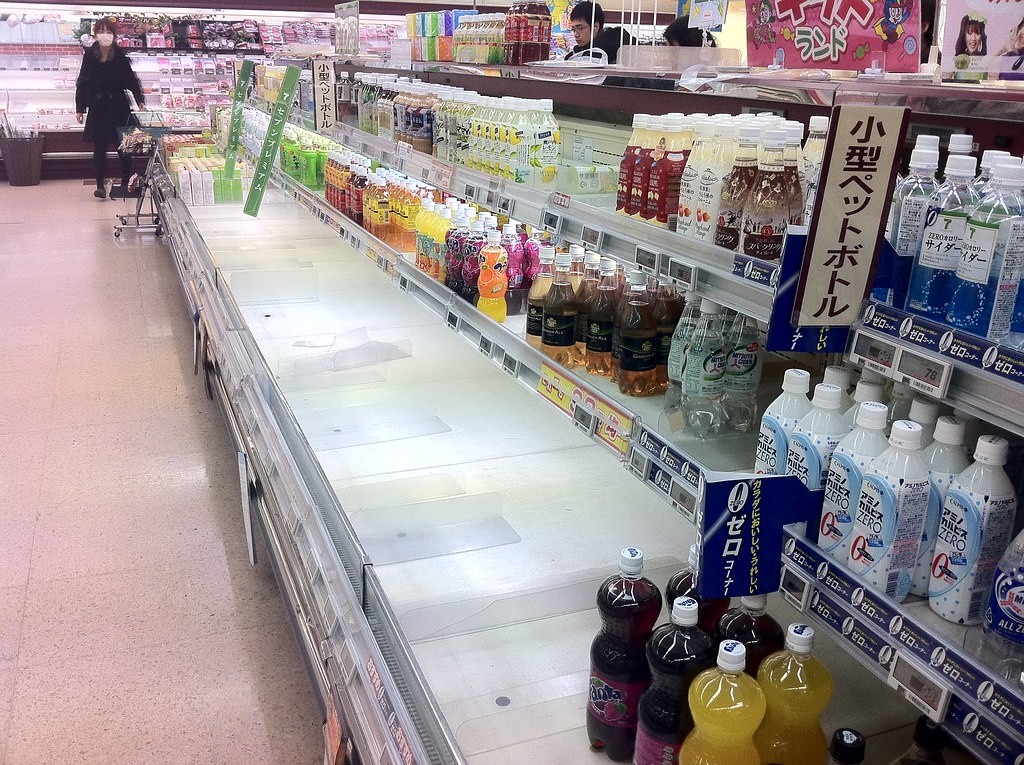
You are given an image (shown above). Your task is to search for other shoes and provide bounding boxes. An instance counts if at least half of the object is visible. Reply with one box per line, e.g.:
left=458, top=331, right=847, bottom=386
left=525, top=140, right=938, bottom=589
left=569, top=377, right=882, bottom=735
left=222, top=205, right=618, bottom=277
left=94, top=189, right=106, bottom=198
left=121, top=179, right=128, bottom=190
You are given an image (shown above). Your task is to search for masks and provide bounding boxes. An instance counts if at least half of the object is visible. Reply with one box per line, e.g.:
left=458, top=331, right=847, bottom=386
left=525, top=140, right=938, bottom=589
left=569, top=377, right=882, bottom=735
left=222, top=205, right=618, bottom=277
left=96, top=34, right=114, bottom=46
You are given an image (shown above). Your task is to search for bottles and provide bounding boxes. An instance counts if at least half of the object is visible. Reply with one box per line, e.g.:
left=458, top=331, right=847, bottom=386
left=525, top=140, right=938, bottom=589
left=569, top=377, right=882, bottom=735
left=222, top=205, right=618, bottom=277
left=334, top=71, right=560, bottom=193
left=634, top=596, right=714, bottom=765
left=664, top=543, right=731, bottom=632
left=280, top=130, right=334, bottom=190
left=755, top=623, right=834, bottom=765
left=676, top=639, right=769, bottom=765
left=585, top=546, right=663, bottom=760
left=888, top=714, right=948, bottom=765
left=871, top=133, right=1024, bottom=341
left=566, top=164, right=620, bottom=194
left=525, top=243, right=764, bottom=439
left=324, top=145, right=442, bottom=255
left=213, top=64, right=285, bottom=166
left=414, top=196, right=552, bottom=324
left=614, top=112, right=831, bottom=263
left=713, top=593, right=785, bottom=681
left=826, top=728, right=868, bottom=765
left=452, top=0, right=552, bottom=65
left=754, top=365, right=1024, bottom=667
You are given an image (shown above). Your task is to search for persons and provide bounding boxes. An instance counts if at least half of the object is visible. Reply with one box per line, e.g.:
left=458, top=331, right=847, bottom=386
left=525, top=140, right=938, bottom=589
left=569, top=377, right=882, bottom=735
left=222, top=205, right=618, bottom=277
left=76, top=20, right=147, bottom=198
left=562, top=2, right=716, bottom=65
left=921, top=17, right=942, bottom=67
left=954, top=14, right=1024, bottom=57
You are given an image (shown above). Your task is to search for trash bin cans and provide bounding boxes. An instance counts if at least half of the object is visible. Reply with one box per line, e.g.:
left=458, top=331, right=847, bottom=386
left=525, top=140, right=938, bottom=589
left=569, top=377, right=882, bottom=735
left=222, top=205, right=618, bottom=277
left=0, top=135, right=44, bottom=186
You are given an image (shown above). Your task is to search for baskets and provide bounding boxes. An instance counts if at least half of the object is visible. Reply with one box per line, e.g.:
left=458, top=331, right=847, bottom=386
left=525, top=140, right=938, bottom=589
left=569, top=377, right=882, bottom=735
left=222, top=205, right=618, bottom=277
left=115, top=110, right=172, bottom=155
left=0, top=136, right=44, bottom=186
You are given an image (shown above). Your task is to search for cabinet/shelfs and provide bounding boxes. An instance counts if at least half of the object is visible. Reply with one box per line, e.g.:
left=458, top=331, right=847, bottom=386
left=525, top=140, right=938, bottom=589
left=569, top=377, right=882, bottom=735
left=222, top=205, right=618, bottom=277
left=0, top=2, right=1024, bottom=765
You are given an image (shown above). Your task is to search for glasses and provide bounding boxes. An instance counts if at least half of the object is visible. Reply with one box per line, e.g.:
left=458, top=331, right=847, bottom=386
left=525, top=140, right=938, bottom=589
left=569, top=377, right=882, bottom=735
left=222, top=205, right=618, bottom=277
left=570, top=25, right=589, bottom=34
